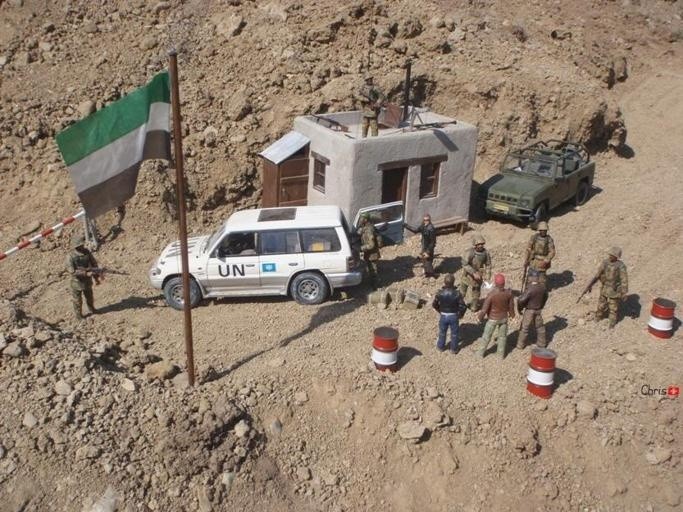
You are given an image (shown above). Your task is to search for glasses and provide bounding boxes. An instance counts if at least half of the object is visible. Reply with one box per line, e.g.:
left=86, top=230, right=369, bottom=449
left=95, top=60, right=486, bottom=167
left=424, top=219, right=429, bottom=221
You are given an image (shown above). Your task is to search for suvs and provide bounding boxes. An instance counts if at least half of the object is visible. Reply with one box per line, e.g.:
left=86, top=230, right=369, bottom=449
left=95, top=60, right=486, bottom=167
left=150, top=201, right=404, bottom=309
left=479, top=139, right=595, bottom=227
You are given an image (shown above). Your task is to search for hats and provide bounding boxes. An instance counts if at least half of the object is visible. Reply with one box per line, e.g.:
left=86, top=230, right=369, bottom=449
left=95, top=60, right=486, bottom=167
left=537, top=222, right=549, bottom=230
left=608, top=247, right=622, bottom=258
left=473, top=235, right=485, bottom=246
left=359, top=212, right=370, bottom=219
left=495, top=274, right=505, bottom=284
left=364, top=73, right=374, bottom=81
left=70, top=237, right=84, bottom=248
left=528, top=271, right=539, bottom=277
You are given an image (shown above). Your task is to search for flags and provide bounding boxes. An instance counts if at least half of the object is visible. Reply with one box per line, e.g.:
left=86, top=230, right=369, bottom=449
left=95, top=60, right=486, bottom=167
left=54, top=66, right=173, bottom=221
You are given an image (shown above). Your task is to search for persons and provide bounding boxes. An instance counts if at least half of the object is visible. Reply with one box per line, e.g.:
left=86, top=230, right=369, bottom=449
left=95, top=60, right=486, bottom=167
left=357, top=211, right=382, bottom=290
left=402, top=215, right=440, bottom=280
left=67, top=236, right=100, bottom=320
left=517, top=270, right=549, bottom=350
left=356, top=76, right=385, bottom=137
left=594, top=246, right=628, bottom=328
left=477, top=274, right=517, bottom=360
left=459, top=235, right=492, bottom=312
left=430, top=273, right=468, bottom=355
left=521, top=221, right=555, bottom=293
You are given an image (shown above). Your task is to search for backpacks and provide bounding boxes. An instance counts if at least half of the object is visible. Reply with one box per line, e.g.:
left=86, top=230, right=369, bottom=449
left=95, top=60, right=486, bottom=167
left=376, top=231, right=384, bottom=249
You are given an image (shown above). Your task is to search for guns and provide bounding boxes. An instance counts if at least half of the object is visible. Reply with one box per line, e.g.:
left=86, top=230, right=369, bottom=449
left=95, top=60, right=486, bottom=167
left=576, top=271, right=606, bottom=304
left=520, top=265, right=527, bottom=293
left=461, top=264, right=484, bottom=285
left=348, top=223, right=391, bottom=246
left=359, top=93, right=387, bottom=115
left=76, top=265, right=130, bottom=287
